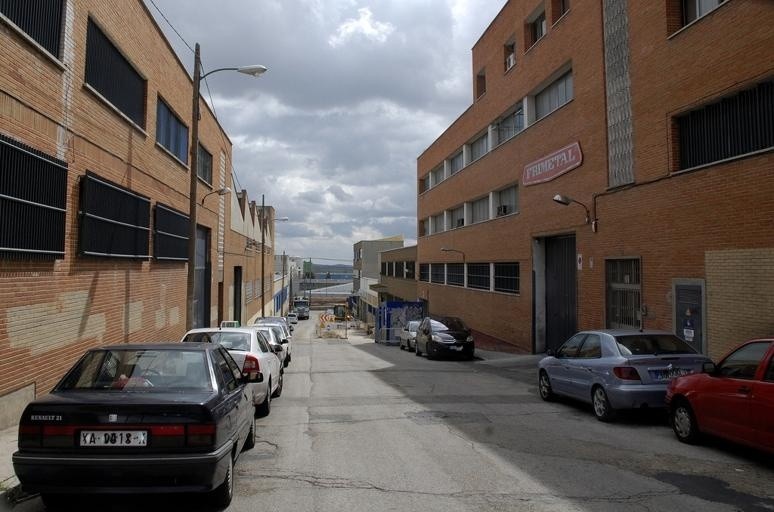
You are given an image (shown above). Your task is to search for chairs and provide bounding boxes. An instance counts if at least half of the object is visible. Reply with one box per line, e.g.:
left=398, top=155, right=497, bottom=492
left=185, top=363, right=207, bottom=387
left=118, top=364, right=140, bottom=380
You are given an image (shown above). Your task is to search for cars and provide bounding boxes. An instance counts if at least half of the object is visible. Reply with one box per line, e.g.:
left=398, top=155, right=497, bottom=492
left=12, top=342, right=264, bottom=507
left=179, top=316, right=294, bottom=416
left=416, top=316, right=474, bottom=359
left=665, top=338, right=774, bottom=452
left=399, top=320, right=422, bottom=350
left=286, top=313, right=298, bottom=324
left=538, top=328, right=716, bottom=421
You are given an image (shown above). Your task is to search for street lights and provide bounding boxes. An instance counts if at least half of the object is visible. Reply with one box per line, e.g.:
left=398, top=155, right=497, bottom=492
left=260, top=195, right=288, bottom=316
left=186, top=43, right=267, bottom=326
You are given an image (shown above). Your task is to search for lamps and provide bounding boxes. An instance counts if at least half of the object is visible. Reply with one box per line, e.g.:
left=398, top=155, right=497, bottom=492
left=442, top=248, right=465, bottom=262
left=552, top=195, right=591, bottom=224
left=202, top=186, right=231, bottom=204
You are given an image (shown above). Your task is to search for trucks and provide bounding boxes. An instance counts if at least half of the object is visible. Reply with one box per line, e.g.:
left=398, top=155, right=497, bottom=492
left=293, top=297, right=310, bottom=320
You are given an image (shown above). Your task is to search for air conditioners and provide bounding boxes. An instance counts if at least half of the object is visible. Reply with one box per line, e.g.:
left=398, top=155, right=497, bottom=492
left=497, top=205, right=506, bottom=215
left=506, top=54, right=515, bottom=70
left=457, top=218, right=464, bottom=227
